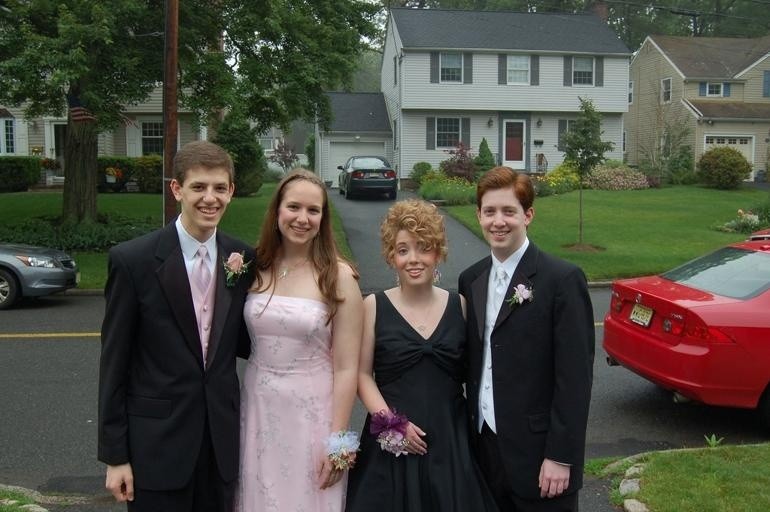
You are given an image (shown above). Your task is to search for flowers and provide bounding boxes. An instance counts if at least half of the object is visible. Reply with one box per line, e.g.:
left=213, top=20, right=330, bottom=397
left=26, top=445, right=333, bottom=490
left=324, top=431, right=362, bottom=473
left=369, top=406, right=410, bottom=460
left=222, top=250, right=255, bottom=290
left=503, top=284, right=535, bottom=310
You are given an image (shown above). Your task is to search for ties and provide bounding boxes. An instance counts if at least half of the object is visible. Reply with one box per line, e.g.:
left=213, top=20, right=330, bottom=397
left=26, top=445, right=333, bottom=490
left=190, top=245, right=212, bottom=302
left=492, top=267, right=507, bottom=315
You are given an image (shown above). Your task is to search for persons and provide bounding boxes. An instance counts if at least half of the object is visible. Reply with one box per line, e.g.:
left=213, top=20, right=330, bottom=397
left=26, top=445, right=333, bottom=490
left=456, top=166, right=596, bottom=511
left=231, top=169, right=364, bottom=512
left=344, top=202, right=500, bottom=512
left=96, top=139, right=261, bottom=511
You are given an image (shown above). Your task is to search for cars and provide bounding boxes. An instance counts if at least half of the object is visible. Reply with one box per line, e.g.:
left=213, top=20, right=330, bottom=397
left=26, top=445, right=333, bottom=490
left=0, top=238, right=81, bottom=309
left=601, top=228, right=770, bottom=443
left=337, top=153, right=399, bottom=200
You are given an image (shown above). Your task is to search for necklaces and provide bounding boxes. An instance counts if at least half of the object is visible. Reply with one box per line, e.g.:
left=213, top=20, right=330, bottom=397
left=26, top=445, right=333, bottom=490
left=277, top=266, right=294, bottom=280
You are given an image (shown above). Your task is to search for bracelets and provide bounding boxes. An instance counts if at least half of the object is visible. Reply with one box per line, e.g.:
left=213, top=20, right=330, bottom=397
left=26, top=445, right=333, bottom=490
left=369, top=407, right=413, bottom=457
left=326, top=429, right=362, bottom=473
